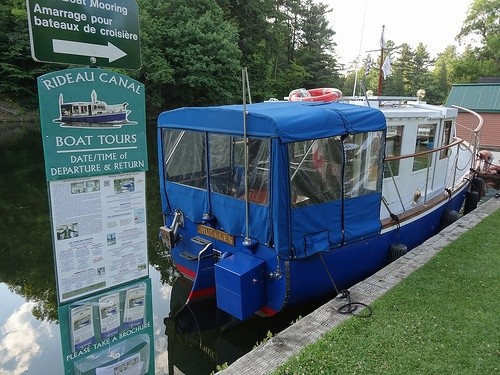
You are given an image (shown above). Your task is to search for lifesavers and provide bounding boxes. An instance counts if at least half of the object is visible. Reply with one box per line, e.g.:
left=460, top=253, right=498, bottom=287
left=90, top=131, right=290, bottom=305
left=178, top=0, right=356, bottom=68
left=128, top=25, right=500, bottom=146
left=288, top=87, right=343, bottom=102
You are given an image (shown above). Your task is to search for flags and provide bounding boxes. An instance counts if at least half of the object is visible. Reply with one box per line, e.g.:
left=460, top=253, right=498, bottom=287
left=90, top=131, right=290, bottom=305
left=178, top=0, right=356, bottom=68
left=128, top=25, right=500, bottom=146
left=365, top=33, right=392, bottom=80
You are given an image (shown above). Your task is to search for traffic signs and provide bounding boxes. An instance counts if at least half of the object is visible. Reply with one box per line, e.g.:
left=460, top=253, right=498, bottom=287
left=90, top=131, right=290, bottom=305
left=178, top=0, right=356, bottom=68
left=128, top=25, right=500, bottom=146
left=26, top=0, right=142, bottom=70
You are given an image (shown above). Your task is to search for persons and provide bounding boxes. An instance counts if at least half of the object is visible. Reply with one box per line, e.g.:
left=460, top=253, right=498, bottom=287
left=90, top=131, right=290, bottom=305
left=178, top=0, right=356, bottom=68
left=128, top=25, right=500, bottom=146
left=476, top=150, right=500, bottom=174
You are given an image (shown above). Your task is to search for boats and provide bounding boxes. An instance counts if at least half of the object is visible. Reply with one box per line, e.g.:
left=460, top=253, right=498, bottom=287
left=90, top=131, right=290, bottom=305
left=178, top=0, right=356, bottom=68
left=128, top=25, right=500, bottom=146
left=158, top=25, right=484, bottom=336
